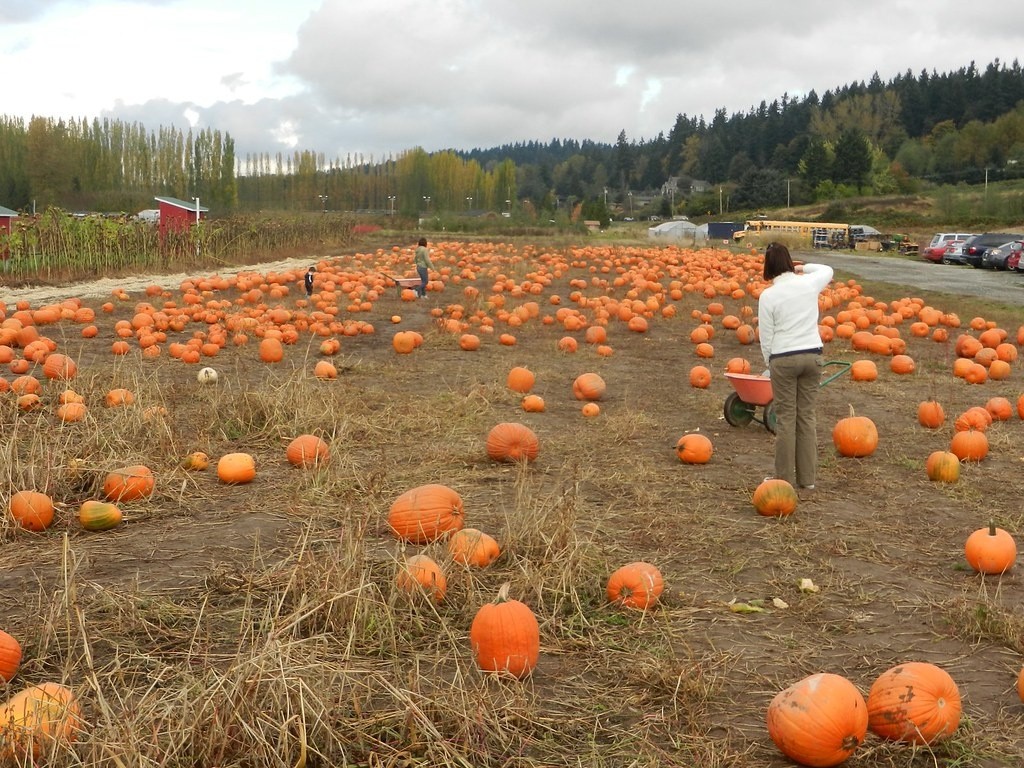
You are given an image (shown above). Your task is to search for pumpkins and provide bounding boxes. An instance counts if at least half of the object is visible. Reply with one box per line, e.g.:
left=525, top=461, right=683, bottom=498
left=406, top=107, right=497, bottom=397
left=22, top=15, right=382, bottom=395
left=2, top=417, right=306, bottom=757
left=0, top=242, right=1024, bottom=768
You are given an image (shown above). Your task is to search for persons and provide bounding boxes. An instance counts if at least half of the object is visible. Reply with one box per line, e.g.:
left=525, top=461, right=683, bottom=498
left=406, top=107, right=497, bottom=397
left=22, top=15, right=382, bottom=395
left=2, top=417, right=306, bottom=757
left=758, top=243, right=834, bottom=490
left=414, top=238, right=434, bottom=299
left=305, top=267, right=316, bottom=299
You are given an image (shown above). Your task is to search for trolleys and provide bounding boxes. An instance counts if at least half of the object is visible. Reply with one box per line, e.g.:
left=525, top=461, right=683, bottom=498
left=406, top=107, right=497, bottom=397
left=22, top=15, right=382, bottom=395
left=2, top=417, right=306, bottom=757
left=723, top=361, right=853, bottom=435
left=380, top=273, right=423, bottom=298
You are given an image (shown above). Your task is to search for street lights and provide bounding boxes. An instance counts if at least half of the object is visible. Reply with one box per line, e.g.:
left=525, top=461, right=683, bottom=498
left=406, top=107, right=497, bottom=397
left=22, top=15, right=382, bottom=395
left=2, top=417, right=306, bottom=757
left=319, top=195, right=328, bottom=214
left=467, top=198, right=472, bottom=208
left=628, top=192, right=633, bottom=213
left=423, top=196, right=431, bottom=210
left=388, top=195, right=396, bottom=216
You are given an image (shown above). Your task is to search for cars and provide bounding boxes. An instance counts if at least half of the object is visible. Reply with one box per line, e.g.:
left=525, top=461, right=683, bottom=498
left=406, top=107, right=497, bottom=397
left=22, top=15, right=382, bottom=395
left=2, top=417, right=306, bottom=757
left=966, top=233, right=1024, bottom=269
left=981, top=241, right=1015, bottom=271
left=651, top=216, right=660, bottom=221
left=1008, top=240, right=1024, bottom=273
left=944, top=244, right=971, bottom=266
left=1018, top=254, right=1024, bottom=269
left=960, top=235, right=981, bottom=269
left=922, top=240, right=965, bottom=265
left=672, top=216, right=689, bottom=221
left=930, top=233, right=983, bottom=248
left=624, top=217, right=633, bottom=221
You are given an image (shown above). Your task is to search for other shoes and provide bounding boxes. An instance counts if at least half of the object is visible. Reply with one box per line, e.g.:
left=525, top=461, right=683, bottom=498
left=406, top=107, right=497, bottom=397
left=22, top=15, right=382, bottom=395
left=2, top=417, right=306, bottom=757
left=305, top=295, right=309, bottom=299
left=412, top=289, right=419, bottom=298
left=802, top=483, right=814, bottom=489
left=421, top=295, right=429, bottom=299
left=764, top=477, right=773, bottom=481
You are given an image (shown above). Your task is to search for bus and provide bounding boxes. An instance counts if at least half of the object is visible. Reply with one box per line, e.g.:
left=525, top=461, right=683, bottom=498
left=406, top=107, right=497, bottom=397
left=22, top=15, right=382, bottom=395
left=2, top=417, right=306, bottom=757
left=733, top=220, right=849, bottom=244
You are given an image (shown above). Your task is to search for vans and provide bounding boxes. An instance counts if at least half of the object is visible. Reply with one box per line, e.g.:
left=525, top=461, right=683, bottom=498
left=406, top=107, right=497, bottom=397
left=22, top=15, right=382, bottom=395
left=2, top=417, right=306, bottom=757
left=849, top=224, right=881, bottom=236
